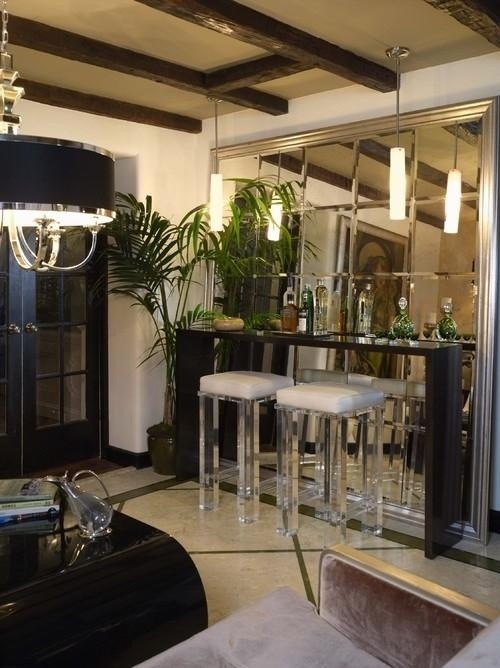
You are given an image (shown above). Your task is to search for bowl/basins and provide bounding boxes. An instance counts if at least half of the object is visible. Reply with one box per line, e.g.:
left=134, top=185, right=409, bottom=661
left=214, top=317, right=245, bottom=331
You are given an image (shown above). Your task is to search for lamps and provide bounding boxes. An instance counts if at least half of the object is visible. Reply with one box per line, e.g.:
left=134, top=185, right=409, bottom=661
left=384, top=47, right=409, bottom=221
left=1, top=0, right=113, bottom=274
left=266, top=149, right=284, bottom=241
left=206, top=96, right=223, bottom=233
left=443, top=121, right=462, bottom=236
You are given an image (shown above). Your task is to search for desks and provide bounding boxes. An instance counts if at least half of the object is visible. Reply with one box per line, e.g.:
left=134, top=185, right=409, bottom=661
left=2, top=500, right=209, bottom=667
left=170, top=322, right=465, bottom=559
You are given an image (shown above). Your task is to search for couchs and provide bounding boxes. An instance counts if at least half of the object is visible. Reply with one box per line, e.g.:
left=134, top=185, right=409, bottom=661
left=130, top=547, right=500, bottom=667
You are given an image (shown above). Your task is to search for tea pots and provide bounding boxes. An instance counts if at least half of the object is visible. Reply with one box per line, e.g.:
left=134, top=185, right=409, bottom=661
left=41, top=468, right=115, bottom=533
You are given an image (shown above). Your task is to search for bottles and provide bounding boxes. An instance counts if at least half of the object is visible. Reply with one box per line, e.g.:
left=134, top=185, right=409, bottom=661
left=392, top=297, right=415, bottom=341
left=435, top=302, right=458, bottom=343
left=280, top=276, right=375, bottom=337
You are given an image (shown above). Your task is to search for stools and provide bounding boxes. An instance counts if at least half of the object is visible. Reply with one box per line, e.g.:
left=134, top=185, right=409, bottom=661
left=194, top=367, right=292, bottom=523
left=290, top=362, right=346, bottom=386
left=345, top=369, right=424, bottom=510
left=274, top=385, right=392, bottom=547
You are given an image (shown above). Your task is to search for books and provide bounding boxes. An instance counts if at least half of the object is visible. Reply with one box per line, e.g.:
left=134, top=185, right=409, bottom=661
left=0, top=476, right=60, bottom=523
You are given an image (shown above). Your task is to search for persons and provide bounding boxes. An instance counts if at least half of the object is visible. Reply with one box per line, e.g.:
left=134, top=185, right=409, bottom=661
left=342, top=256, right=398, bottom=377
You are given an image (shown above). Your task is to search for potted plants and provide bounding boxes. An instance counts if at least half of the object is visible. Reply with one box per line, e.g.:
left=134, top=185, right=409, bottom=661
left=98, top=179, right=193, bottom=477
